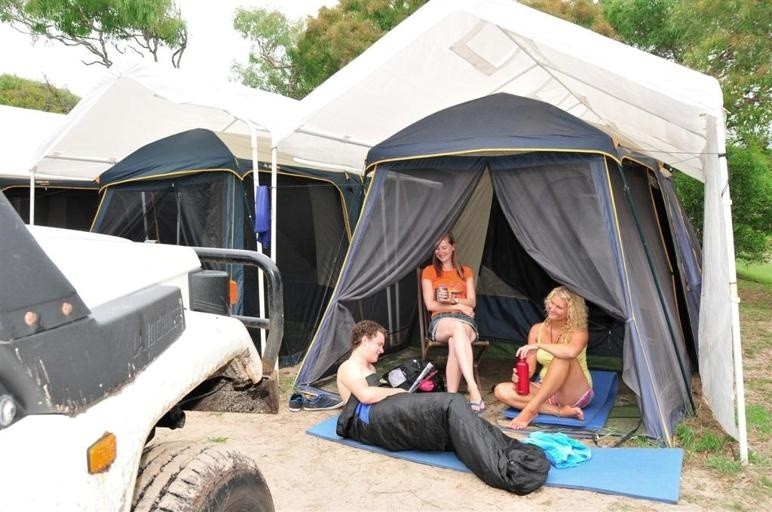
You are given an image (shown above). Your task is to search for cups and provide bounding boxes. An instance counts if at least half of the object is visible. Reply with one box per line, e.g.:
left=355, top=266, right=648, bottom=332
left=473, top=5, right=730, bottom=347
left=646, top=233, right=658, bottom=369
left=434, top=287, right=449, bottom=302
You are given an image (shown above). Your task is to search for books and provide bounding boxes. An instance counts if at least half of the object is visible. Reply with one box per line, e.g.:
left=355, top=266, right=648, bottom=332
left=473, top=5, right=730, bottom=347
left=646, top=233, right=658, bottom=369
left=408, top=362, right=438, bottom=393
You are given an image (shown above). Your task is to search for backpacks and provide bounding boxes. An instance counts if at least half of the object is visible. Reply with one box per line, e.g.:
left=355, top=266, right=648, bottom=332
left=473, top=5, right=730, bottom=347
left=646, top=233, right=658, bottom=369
left=380, top=358, right=444, bottom=392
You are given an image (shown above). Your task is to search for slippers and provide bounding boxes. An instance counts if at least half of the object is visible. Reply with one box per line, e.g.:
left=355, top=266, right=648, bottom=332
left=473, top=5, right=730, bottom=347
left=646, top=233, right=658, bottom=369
left=469, top=398, right=485, bottom=413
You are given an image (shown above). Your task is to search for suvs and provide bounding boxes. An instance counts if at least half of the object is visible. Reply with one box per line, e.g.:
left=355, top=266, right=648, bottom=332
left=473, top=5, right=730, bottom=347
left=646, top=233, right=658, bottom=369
left=0, top=187, right=285, bottom=512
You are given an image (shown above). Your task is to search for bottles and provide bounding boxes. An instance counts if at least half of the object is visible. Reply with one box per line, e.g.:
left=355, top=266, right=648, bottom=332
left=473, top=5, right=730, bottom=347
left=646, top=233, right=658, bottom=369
left=517, top=358, right=530, bottom=394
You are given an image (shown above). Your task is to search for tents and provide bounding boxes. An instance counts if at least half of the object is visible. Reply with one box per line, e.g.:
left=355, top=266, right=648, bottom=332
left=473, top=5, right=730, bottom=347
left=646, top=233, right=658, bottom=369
left=89, top=129, right=371, bottom=369
left=292, top=93, right=702, bottom=447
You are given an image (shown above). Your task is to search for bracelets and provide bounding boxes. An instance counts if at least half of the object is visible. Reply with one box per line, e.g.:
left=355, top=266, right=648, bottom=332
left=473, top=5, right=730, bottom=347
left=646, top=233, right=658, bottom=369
left=452, top=296, right=458, bottom=305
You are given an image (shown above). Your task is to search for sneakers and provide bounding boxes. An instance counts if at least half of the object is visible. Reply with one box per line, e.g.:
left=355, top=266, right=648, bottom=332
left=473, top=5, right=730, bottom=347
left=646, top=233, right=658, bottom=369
left=289, top=391, right=303, bottom=412
left=304, top=393, right=343, bottom=411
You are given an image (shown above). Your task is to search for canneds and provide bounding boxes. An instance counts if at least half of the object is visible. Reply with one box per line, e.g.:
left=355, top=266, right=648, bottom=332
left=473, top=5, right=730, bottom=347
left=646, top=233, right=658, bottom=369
left=450, top=289, right=464, bottom=305
left=436, top=287, right=449, bottom=304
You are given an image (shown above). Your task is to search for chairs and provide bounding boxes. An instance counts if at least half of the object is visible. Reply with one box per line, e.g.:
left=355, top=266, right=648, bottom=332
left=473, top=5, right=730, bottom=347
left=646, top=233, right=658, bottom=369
left=416, top=266, right=490, bottom=394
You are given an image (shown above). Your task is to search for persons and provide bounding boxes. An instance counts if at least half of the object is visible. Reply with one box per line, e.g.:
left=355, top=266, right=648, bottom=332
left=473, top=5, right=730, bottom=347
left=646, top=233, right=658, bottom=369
left=421, top=232, right=486, bottom=415
left=337, top=320, right=409, bottom=404
left=494, top=285, right=594, bottom=430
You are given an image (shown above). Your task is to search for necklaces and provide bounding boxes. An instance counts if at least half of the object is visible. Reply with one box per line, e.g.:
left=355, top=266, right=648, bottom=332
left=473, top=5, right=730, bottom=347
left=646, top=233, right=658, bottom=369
left=550, top=323, right=559, bottom=344
